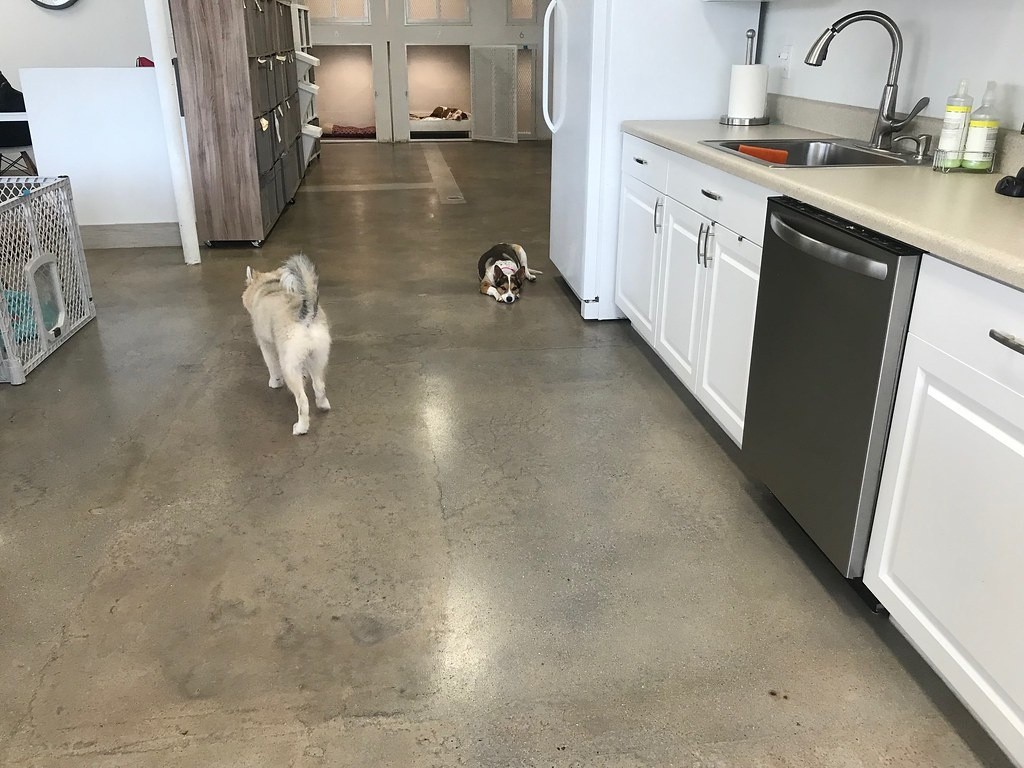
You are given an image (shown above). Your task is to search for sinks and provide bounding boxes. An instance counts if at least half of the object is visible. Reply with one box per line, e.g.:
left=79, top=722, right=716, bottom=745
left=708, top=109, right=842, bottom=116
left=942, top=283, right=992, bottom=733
left=696, top=136, right=931, bottom=169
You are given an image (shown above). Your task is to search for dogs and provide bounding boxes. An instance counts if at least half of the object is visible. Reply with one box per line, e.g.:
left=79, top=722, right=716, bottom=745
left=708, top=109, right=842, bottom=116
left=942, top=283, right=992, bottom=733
left=241, top=252, right=331, bottom=436
left=430, top=106, right=468, bottom=121
left=475, top=242, right=543, bottom=305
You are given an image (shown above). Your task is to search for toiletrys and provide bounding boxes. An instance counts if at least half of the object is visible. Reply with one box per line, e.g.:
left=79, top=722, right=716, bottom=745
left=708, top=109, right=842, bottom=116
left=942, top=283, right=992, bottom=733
left=935, top=75, right=974, bottom=168
left=962, top=79, right=1001, bottom=171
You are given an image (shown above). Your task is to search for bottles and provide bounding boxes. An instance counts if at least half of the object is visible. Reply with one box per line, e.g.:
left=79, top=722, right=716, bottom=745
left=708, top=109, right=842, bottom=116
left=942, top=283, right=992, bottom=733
left=934, top=80, right=974, bottom=168
left=964, top=81, right=997, bottom=170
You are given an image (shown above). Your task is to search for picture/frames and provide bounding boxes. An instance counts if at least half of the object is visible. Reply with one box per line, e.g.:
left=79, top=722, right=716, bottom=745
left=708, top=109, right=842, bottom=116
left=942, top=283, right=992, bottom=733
left=31, top=0, right=78, bottom=10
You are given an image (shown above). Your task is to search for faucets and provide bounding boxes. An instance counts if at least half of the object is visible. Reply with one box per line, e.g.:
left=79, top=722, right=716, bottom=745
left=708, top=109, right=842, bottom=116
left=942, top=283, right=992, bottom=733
left=803, top=9, right=904, bottom=150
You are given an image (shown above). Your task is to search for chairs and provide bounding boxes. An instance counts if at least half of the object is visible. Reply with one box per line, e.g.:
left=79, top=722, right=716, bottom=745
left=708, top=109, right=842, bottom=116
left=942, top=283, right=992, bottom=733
left=0, top=112, right=38, bottom=176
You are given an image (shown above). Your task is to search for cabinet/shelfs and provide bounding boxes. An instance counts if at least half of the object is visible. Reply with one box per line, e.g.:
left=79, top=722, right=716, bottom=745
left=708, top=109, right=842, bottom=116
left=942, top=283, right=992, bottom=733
left=293, top=0, right=551, bottom=144
left=610, top=130, right=784, bottom=453
left=168, top=0, right=306, bottom=250
left=862, top=250, right=1024, bottom=768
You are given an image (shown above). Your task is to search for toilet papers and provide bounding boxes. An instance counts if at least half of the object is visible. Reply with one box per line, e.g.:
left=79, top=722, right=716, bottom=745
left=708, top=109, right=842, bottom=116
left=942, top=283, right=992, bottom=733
left=727, top=62, right=768, bottom=119
